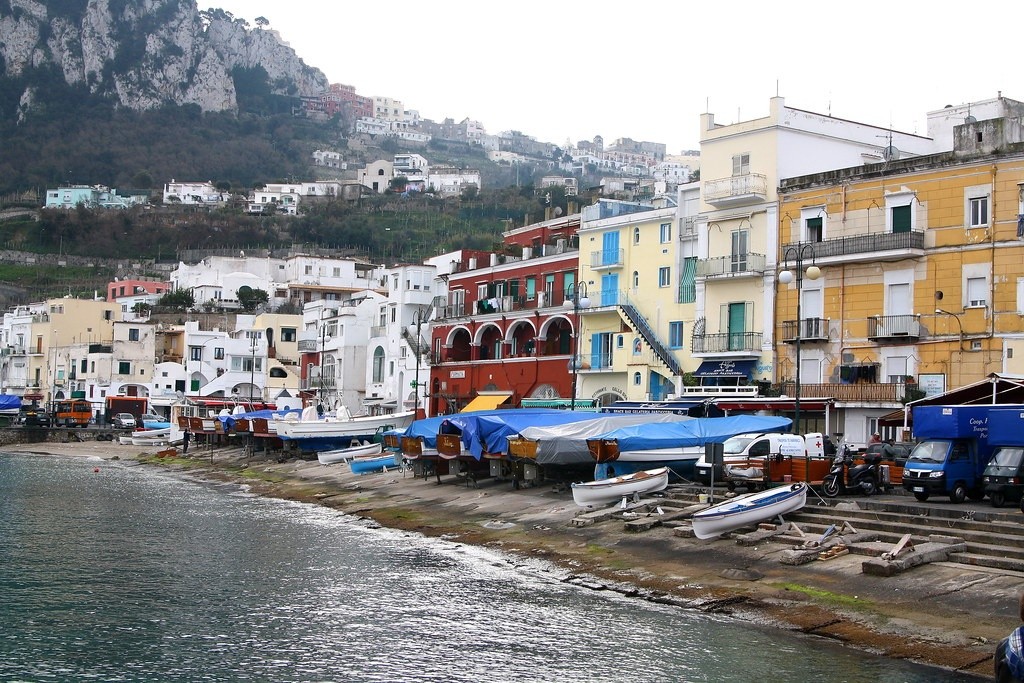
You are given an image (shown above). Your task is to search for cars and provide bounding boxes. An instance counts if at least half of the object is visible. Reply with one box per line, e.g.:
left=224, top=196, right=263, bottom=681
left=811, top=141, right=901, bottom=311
left=17, top=410, right=51, bottom=428
left=862, top=442, right=918, bottom=467
left=114, top=413, right=158, bottom=430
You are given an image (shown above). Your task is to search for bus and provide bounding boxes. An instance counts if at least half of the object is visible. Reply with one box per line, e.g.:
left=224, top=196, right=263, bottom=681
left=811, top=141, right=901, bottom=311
left=53, top=400, right=92, bottom=428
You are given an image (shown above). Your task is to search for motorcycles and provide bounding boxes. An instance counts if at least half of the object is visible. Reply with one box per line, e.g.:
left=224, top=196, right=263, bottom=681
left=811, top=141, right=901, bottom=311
left=983, top=446, right=1024, bottom=507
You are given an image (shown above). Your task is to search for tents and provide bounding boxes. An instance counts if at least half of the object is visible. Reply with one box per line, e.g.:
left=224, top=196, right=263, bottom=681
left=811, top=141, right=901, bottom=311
left=586, top=416, right=793, bottom=506
left=904, top=373, right=1024, bottom=447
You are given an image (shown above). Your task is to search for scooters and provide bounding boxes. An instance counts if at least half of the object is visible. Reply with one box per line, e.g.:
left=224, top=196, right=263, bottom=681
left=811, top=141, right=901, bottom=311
left=822, top=445, right=882, bottom=498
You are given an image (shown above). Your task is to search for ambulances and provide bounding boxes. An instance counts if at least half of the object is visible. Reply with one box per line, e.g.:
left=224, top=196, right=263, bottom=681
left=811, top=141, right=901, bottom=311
left=693, top=431, right=824, bottom=487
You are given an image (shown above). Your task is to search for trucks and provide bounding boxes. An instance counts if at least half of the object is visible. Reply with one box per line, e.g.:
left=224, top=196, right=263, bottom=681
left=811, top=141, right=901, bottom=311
left=899, top=403, right=1024, bottom=504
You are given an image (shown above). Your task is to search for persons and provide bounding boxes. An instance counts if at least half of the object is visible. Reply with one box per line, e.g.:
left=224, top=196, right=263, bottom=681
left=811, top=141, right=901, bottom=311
left=524, top=339, right=534, bottom=356
left=636, top=341, right=641, bottom=352
left=183, top=428, right=193, bottom=454
left=481, top=296, right=489, bottom=310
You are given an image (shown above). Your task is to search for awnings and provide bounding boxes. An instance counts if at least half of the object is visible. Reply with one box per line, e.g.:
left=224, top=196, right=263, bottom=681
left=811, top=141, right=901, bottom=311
left=692, top=358, right=758, bottom=377
left=601, top=397, right=707, bottom=409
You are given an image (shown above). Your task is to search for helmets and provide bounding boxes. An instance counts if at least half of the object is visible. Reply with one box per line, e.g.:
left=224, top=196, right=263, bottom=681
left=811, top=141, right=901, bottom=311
left=844, top=456, right=854, bottom=466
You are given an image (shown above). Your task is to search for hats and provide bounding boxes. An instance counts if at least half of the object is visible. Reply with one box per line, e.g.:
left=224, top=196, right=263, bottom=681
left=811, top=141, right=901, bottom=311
left=874, top=431, right=880, bottom=435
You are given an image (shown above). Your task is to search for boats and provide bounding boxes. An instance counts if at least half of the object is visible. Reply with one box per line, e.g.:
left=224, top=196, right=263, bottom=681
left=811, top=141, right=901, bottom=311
left=381, top=407, right=793, bottom=482
left=178, top=406, right=336, bottom=437
left=275, top=409, right=416, bottom=453
left=318, top=442, right=381, bottom=464
left=571, top=465, right=670, bottom=505
left=349, top=455, right=400, bottom=473
left=690, top=480, right=807, bottom=539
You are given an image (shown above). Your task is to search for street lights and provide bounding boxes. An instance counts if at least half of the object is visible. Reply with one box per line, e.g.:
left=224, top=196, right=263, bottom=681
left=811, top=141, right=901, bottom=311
left=249, top=336, right=259, bottom=408
left=410, top=310, right=429, bottom=420
left=317, top=324, right=332, bottom=404
left=779, top=243, right=821, bottom=436
left=51, top=330, right=58, bottom=415
left=563, top=280, right=590, bottom=411
left=199, top=336, right=219, bottom=400
